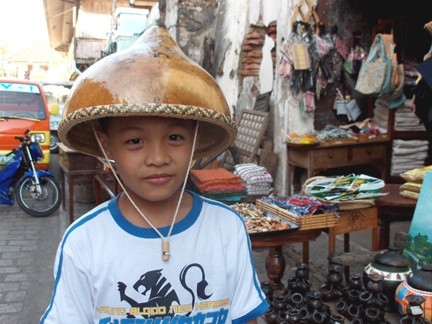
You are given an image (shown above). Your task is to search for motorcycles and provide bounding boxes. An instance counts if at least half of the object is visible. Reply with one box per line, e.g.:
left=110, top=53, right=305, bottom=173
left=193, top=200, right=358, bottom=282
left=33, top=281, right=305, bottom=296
left=0, top=129, right=62, bottom=217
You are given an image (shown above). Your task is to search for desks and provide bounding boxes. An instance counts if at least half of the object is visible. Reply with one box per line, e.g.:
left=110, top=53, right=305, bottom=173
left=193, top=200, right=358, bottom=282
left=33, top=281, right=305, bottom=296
left=228, top=184, right=432, bottom=290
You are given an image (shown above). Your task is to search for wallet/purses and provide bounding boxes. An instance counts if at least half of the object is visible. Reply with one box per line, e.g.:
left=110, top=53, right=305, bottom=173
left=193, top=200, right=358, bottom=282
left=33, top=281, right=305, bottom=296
left=278, top=21, right=368, bottom=121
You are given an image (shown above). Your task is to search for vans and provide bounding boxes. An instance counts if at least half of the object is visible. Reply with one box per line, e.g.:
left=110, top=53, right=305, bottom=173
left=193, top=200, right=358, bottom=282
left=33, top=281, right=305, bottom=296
left=40, top=81, right=73, bottom=153
left=0, top=79, right=51, bottom=183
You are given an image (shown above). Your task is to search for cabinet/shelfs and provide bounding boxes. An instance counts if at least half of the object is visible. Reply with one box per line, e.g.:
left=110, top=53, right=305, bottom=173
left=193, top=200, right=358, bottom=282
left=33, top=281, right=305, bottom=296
left=57, top=142, right=118, bottom=225
left=285, top=84, right=432, bottom=196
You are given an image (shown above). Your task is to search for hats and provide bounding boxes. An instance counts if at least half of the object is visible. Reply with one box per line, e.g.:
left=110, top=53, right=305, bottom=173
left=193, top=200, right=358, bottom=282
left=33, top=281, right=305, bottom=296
left=57, top=25, right=236, bottom=169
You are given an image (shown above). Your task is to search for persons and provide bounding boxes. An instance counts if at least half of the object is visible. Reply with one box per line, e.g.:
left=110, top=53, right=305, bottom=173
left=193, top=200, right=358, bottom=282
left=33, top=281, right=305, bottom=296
left=410, top=21, right=432, bottom=166
left=39, top=26, right=271, bottom=324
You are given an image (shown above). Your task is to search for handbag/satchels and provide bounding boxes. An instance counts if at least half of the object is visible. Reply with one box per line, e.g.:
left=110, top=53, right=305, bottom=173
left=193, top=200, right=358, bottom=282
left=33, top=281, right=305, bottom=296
left=352, top=33, right=407, bottom=108
